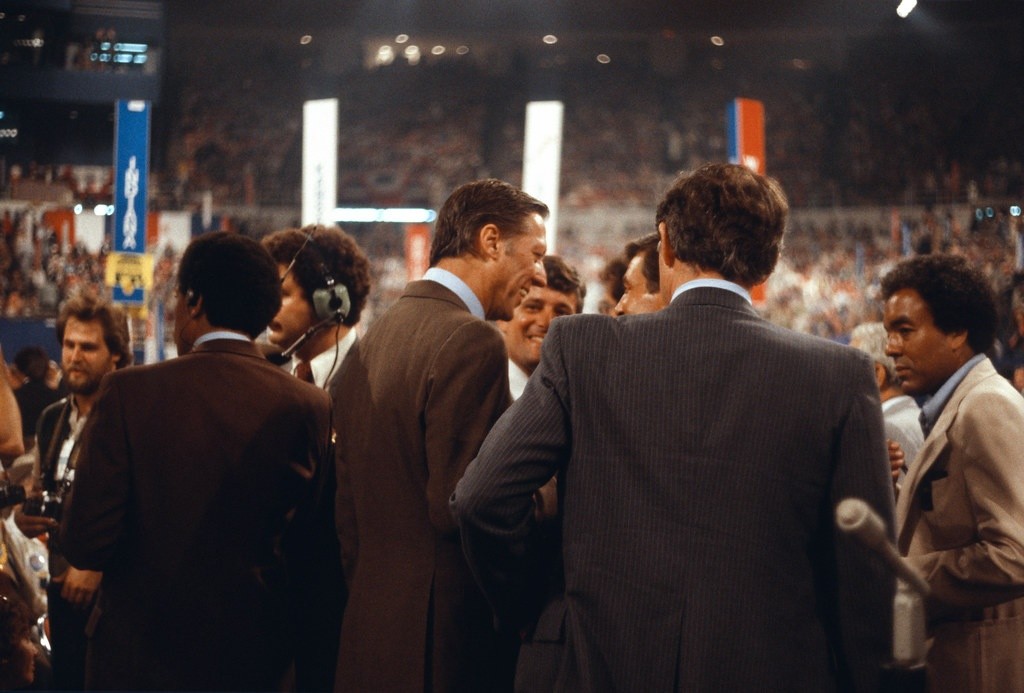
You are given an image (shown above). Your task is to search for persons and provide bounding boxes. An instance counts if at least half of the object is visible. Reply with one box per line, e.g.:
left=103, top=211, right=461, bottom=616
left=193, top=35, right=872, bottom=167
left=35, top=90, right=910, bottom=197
left=493, top=257, right=587, bottom=405
left=14, top=304, right=132, bottom=693
left=334, top=180, right=546, bottom=693
left=452, top=163, right=897, bottom=690
left=599, top=258, right=628, bottom=322
left=616, top=234, right=660, bottom=320
left=883, top=257, right=1024, bottom=693
left=53, top=230, right=334, bottom=693
left=0, top=52, right=1024, bottom=693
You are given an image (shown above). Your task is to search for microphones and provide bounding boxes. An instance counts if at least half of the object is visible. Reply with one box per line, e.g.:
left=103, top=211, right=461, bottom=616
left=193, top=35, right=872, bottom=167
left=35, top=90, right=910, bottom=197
left=280, top=226, right=316, bottom=283
left=835, top=496, right=938, bottom=607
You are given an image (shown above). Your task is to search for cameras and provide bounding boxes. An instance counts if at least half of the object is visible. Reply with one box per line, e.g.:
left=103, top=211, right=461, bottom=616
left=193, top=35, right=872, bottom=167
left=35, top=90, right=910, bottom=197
left=22, top=494, right=62, bottom=518
left=0, top=485, right=26, bottom=510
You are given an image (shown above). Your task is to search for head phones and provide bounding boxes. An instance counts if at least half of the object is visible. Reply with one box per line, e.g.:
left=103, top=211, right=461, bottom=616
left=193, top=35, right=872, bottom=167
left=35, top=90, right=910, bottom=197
left=299, top=230, right=350, bottom=325
left=265, top=317, right=331, bottom=364
left=186, top=233, right=231, bottom=306
